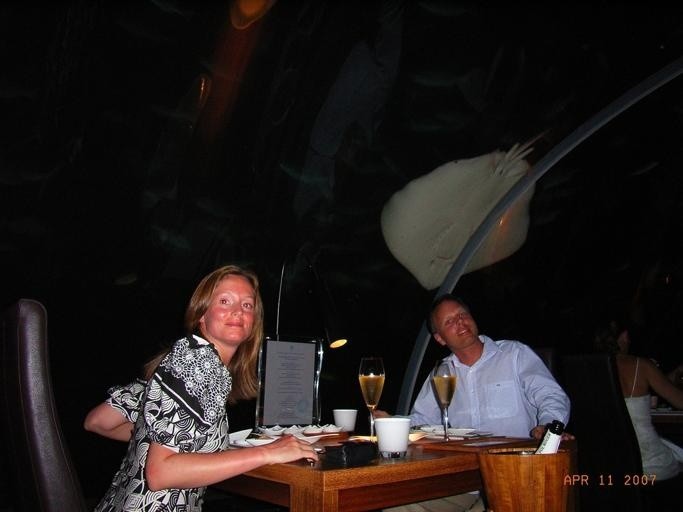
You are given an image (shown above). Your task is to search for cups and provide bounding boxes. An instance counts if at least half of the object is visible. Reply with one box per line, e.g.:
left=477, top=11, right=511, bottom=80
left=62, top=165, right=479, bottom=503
left=331, top=408, right=357, bottom=437
left=373, top=418, right=411, bottom=459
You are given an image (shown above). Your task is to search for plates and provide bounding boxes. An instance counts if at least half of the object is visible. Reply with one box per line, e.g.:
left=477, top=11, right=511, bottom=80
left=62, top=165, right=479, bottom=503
left=420, top=425, right=476, bottom=437
left=229, top=438, right=275, bottom=449
left=227, top=429, right=252, bottom=445
left=257, top=424, right=345, bottom=436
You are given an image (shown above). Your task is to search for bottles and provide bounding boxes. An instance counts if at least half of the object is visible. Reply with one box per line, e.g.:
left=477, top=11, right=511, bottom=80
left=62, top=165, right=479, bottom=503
left=534, top=420, right=565, bottom=455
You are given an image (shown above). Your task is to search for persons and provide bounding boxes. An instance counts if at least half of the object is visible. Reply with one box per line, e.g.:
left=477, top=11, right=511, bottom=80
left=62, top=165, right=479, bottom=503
left=605, top=319, right=681, bottom=484
left=80, top=264, right=321, bottom=511
left=364, top=292, right=576, bottom=457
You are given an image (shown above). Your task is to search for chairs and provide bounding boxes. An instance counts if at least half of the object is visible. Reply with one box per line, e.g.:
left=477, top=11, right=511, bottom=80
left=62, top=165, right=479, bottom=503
left=16, top=298, right=87, bottom=512
left=505, top=333, right=552, bottom=372
left=586, top=352, right=683, bottom=512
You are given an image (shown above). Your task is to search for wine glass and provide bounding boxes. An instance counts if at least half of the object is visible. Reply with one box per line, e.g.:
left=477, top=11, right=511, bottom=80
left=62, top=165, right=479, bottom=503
left=432, top=359, right=459, bottom=441
left=358, top=355, right=385, bottom=446
